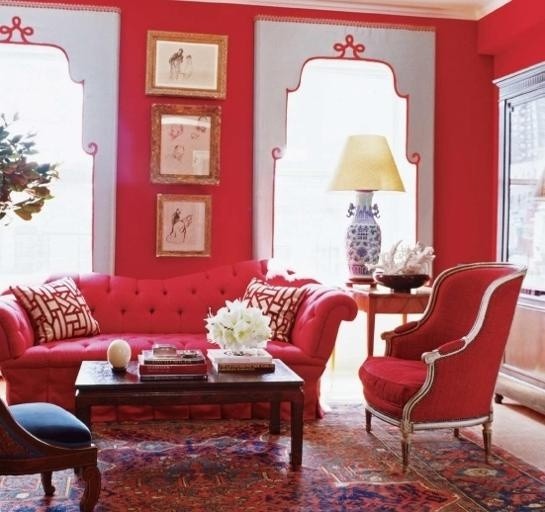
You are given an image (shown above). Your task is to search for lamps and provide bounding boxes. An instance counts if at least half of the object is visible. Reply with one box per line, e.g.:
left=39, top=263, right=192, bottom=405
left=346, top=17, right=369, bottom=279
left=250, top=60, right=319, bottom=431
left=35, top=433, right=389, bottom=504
left=328, top=135, right=405, bottom=288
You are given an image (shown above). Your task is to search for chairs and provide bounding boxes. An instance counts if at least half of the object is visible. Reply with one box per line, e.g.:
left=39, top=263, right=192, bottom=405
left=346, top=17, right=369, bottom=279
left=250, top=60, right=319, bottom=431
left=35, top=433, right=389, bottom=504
left=364, top=259, right=527, bottom=473
left=0, top=395, right=102, bottom=512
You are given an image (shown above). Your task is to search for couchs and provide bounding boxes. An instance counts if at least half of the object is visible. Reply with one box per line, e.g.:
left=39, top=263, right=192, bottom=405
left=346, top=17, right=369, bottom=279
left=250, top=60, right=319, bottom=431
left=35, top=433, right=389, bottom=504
left=1, top=263, right=358, bottom=422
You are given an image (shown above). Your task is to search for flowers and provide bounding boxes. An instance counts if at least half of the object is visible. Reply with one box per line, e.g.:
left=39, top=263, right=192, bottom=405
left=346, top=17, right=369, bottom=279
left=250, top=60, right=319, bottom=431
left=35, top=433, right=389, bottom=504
left=203, top=298, right=275, bottom=356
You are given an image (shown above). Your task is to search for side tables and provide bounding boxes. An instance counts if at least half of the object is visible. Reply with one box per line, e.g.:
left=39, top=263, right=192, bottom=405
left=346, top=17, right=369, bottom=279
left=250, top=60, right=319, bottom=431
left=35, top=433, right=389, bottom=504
left=329, top=286, right=432, bottom=371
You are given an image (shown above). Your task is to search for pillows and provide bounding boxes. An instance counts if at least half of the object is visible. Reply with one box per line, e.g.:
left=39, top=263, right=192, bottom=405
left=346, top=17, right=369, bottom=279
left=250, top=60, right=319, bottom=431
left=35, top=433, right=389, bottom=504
left=238, top=277, right=311, bottom=343
left=7, top=275, right=100, bottom=345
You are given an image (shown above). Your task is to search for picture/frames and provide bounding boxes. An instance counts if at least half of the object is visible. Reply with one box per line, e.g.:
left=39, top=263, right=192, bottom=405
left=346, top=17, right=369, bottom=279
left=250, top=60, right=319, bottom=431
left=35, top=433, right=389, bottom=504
left=155, top=192, right=211, bottom=258
left=147, top=103, right=222, bottom=188
left=143, top=28, right=229, bottom=100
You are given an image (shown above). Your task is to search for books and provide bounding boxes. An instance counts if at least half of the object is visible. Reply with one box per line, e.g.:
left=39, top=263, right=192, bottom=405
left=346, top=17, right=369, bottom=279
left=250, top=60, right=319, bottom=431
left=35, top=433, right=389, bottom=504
left=135, top=343, right=276, bottom=382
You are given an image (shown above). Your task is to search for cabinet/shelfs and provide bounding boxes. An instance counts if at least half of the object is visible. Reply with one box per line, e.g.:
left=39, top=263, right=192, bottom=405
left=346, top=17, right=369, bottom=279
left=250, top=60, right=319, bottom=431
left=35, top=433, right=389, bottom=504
left=490, top=62, right=545, bottom=423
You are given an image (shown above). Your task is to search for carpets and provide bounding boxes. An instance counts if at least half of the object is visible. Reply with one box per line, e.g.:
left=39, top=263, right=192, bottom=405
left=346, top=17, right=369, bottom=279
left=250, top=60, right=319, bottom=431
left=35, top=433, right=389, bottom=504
left=0, top=401, right=545, bottom=512
